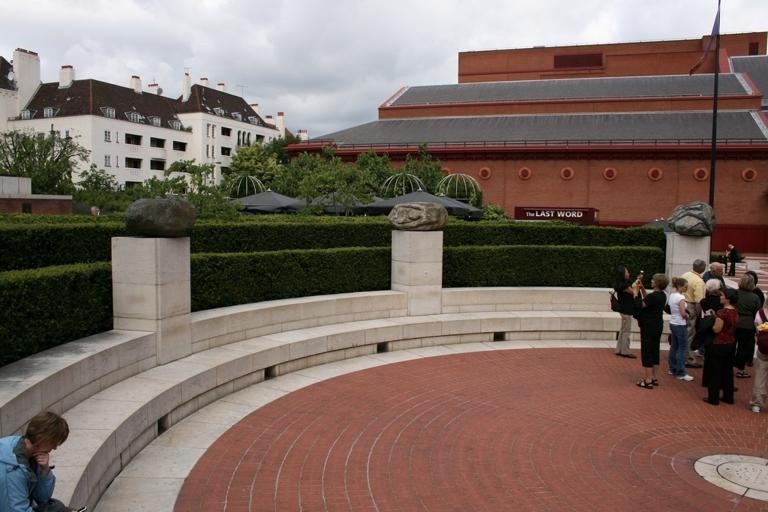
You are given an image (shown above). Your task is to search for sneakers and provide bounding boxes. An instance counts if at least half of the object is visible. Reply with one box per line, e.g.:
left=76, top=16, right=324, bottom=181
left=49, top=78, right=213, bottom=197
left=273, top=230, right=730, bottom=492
left=668, top=370, right=694, bottom=381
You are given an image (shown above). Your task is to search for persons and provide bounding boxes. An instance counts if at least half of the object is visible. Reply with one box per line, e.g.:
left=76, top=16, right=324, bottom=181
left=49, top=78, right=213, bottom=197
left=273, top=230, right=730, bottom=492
left=614, top=244, right=768, bottom=412
left=0, top=410, right=79, bottom=512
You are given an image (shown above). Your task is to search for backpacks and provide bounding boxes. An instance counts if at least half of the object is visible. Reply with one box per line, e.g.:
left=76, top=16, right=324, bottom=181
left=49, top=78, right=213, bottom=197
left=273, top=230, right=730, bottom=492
left=609, top=290, right=622, bottom=311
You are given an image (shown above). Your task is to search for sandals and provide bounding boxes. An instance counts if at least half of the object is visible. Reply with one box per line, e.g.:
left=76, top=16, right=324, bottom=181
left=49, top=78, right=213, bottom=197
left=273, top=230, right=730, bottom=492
left=637, top=379, right=658, bottom=389
left=737, top=372, right=751, bottom=378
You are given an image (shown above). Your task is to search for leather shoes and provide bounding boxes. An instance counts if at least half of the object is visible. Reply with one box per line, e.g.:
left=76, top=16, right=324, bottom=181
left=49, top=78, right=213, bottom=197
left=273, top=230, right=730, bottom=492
left=685, top=363, right=702, bottom=368
left=616, top=353, right=636, bottom=358
